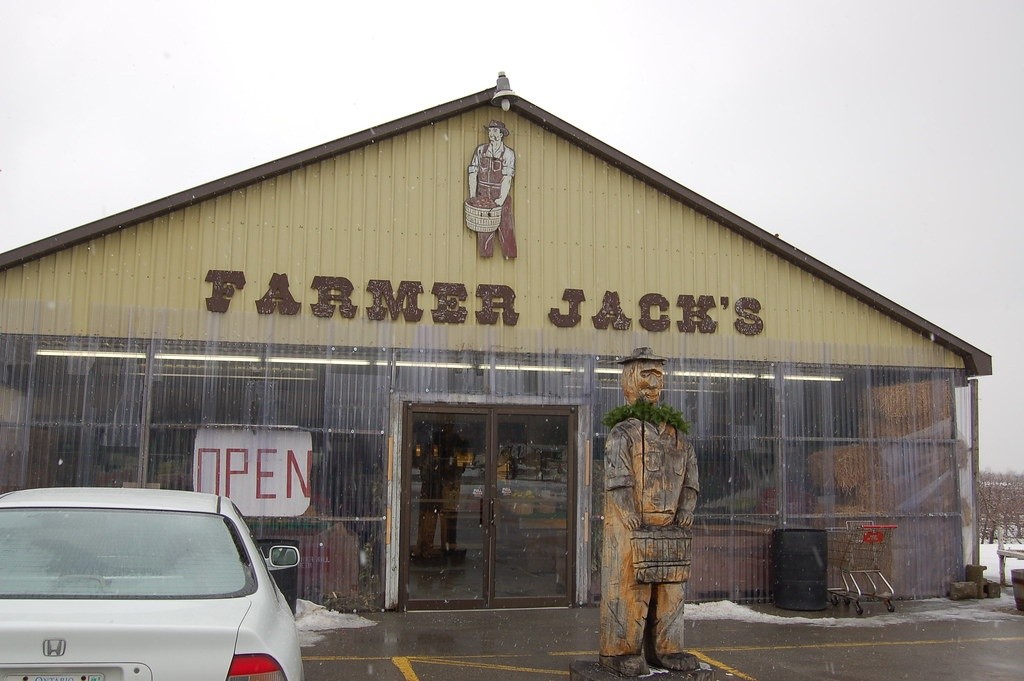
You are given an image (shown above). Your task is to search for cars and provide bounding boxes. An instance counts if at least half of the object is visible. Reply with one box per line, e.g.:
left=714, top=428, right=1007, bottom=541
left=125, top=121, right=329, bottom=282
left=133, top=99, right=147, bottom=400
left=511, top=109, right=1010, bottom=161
left=0, top=486, right=306, bottom=681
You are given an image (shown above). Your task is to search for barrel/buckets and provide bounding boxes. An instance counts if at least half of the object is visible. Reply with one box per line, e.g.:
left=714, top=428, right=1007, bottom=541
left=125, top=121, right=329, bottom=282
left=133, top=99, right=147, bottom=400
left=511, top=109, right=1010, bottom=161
left=1011, top=569, right=1024, bottom=611
left=770, top=528, right=829, bottom=610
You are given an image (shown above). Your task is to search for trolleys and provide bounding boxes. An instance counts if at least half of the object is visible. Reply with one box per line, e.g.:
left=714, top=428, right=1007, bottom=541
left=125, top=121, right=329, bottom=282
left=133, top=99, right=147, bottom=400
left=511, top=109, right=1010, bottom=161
left=824, top=519, right=898, bottom=615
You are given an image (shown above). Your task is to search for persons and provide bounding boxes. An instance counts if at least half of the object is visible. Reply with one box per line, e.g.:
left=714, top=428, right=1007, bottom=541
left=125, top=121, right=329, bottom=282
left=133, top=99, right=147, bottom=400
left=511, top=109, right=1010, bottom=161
left=601, top=346, right=699, bottom=676
